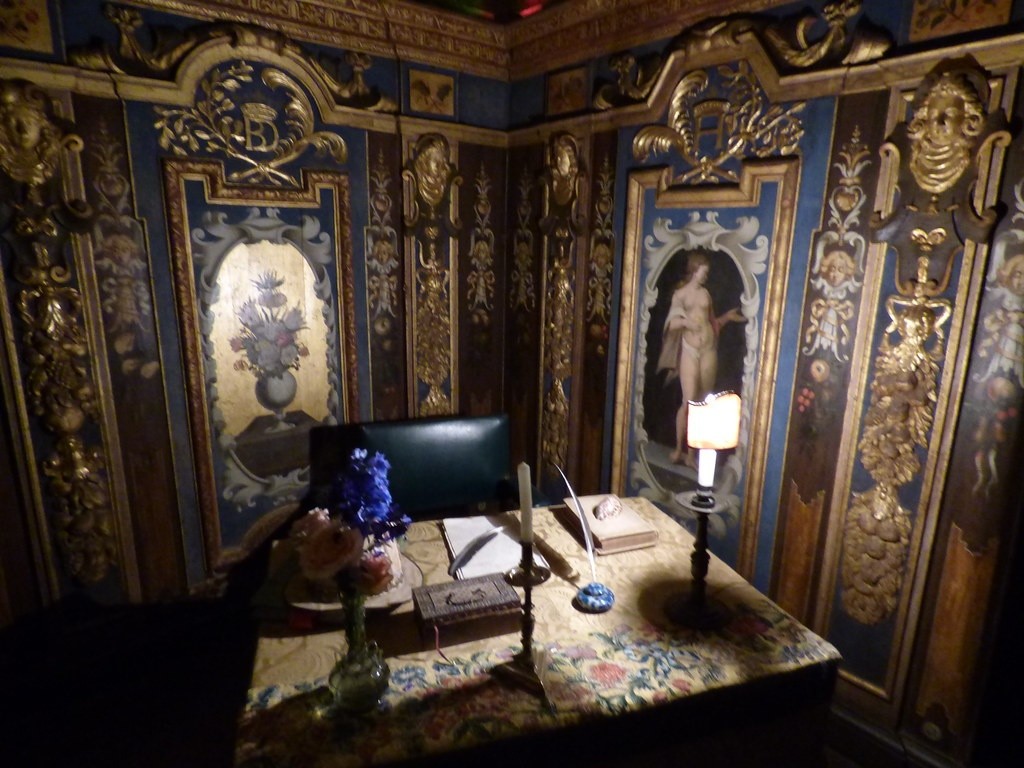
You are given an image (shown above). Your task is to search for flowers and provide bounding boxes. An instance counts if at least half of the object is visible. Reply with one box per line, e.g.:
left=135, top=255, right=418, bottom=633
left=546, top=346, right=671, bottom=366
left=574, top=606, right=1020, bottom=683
left=261, top=449, right=412, bottom=595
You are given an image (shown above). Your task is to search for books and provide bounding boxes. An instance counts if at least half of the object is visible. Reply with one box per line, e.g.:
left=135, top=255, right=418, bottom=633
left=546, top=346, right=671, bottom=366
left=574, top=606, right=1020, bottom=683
left=562, top=493, right=659, bottom=555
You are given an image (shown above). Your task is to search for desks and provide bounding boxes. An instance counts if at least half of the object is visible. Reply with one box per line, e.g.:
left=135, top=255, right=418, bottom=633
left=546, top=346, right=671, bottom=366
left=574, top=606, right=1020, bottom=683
left=232, top=498, right=841, bottom=768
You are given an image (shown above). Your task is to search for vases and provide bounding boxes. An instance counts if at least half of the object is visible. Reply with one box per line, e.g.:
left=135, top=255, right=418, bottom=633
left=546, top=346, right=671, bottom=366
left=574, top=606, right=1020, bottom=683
left=326, top=582, right=392, bottom=711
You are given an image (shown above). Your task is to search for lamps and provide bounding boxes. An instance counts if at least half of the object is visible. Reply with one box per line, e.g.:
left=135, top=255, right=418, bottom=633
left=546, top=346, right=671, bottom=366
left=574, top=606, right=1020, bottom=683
left=664, top=390, right=741, bottom=628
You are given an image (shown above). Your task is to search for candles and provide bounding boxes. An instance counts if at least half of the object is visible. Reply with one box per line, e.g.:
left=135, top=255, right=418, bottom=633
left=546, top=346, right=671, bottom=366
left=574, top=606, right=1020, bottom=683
left=515, top=459, right=534, bottom=542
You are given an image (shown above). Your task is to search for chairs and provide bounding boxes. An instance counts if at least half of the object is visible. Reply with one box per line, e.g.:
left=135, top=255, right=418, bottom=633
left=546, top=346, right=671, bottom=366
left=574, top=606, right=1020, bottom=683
left=307, top=414, right=545, bottom=522
